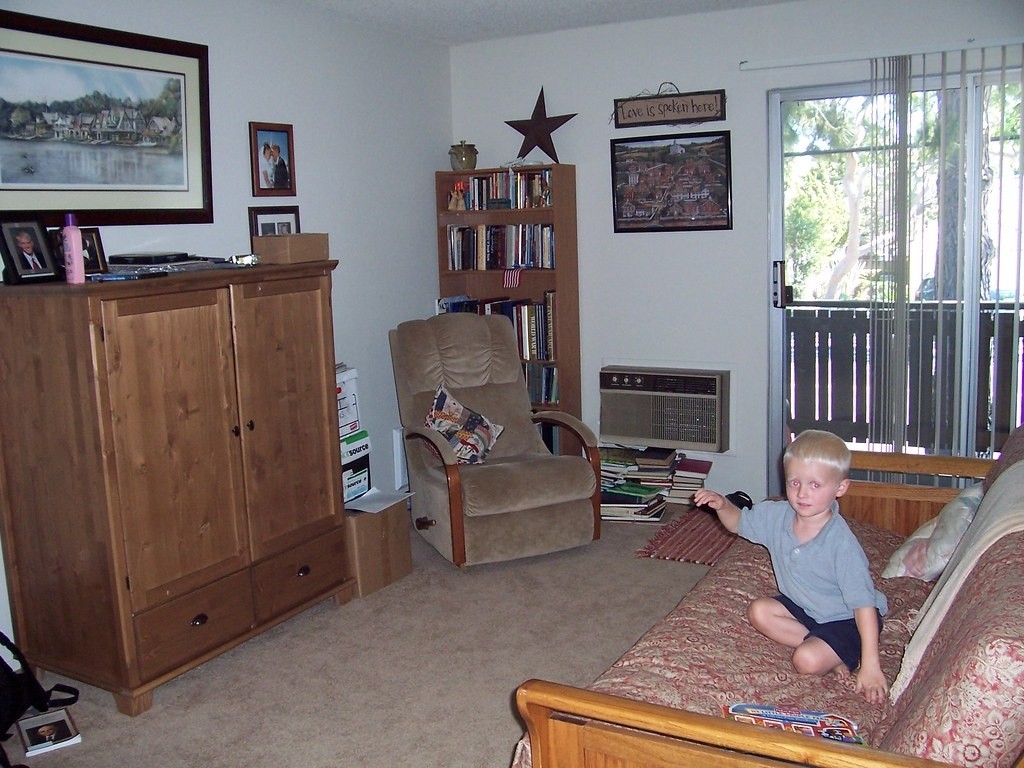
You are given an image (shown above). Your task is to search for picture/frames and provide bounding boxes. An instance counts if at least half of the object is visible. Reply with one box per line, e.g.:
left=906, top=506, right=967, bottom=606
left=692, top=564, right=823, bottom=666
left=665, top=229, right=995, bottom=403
left=249, top=121, right=297, bottom=197
left=49, top=228, right=109, bottom=275
left=0, top=218, right=67, bottom=283
left=611, top=130, right=733, bottom=234
left=248, top=206, right=300, bottom=255
left=0, top=9, right=214, bottom=227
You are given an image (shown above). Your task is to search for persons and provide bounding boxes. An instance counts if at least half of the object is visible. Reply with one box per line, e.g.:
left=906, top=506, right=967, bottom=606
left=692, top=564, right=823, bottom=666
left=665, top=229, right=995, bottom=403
left=694, top=430, right=890, bottom=704
left=279, top=225, right=288, bottom=235
left=259, top=141, right=289, bottom=188
left=81, top=237, right=100, bottom=270
left=16, top=231, right=47, bottom=270
left=36, top=726, right=70, bottom=743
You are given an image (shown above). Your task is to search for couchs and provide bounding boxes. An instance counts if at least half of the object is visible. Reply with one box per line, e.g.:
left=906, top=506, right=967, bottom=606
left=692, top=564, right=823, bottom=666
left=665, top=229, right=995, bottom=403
left=389, top=312, right=603, bottom=570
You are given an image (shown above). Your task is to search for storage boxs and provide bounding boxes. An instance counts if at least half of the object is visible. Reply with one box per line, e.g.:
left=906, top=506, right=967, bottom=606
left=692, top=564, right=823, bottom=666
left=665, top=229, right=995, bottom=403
left=253, top=232, right=330, bottom=263
left=336, top=368, right=412, bottom=600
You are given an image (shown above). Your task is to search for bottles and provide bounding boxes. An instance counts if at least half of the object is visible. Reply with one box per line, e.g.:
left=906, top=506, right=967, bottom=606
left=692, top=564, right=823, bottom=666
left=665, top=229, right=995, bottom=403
left=62, top=213, right=85, bottom=284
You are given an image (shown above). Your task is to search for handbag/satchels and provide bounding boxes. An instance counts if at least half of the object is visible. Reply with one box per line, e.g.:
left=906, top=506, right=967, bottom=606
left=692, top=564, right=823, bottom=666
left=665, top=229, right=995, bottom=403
left=0, top=631, right=79, bottom=732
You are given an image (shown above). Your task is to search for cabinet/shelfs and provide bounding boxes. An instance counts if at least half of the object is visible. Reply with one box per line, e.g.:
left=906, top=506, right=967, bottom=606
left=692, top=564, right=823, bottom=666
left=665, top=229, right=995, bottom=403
left=0, top=261, right=357, bottom=716
left=435, top=163, right=583, bottom=456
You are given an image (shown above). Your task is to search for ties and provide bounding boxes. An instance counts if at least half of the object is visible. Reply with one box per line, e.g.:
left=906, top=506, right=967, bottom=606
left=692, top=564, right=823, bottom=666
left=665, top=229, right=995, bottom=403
left=32, top=258, right=40, bottom=270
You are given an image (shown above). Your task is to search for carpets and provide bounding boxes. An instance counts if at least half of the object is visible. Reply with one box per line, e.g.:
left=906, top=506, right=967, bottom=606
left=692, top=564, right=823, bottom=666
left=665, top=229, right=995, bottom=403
left=636, top=508, right=737, bottom=566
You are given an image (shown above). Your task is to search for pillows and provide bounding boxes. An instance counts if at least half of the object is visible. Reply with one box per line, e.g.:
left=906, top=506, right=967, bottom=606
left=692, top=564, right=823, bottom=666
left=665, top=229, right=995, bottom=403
left=423, top=384, right=505, bottom=464
left=882, top=481, right=985, bottom=580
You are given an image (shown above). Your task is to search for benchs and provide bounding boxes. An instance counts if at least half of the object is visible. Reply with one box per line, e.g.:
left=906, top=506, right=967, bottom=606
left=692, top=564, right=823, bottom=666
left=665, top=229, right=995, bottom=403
left=512, top=422, right=1024, bottom=768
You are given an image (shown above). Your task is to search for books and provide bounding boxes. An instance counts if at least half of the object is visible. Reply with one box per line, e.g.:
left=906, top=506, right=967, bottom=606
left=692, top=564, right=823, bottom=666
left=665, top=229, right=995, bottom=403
left=16, top=708, right=82, bottom=758
left=447, top=170, right=553, bottom=210
left=446, top=223, right=555, bottom=270
left=521, top=362, right=560, bottom=403
left=435, top=290, right=558, bottom=362
left=597, top=445, right=713, bottom=523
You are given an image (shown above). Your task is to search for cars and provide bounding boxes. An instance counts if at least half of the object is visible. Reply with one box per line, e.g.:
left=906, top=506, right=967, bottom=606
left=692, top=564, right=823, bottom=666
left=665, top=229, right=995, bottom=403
left=913, top=274, right=936, bottom=301
